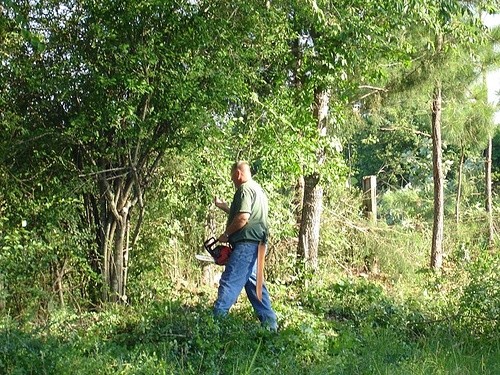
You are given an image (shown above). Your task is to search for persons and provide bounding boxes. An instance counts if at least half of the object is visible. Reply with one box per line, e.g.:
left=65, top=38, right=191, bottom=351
left=210, top=162, right=277, bottom=332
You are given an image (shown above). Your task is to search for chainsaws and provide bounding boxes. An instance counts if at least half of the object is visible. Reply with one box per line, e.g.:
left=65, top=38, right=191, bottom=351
left=195, top=237, right=232, bottom=266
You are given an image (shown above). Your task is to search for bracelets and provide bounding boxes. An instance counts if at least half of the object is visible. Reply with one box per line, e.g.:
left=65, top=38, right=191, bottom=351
left=224, top=232, right=230, bottom=238
